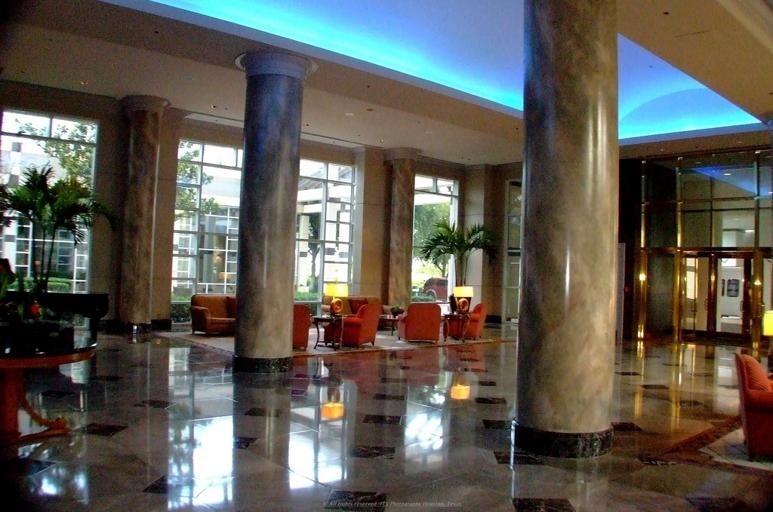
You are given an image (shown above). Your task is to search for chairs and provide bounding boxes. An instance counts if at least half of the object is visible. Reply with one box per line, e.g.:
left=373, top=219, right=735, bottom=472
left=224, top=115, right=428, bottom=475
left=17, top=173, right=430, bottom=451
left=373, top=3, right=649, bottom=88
left=290, top=342, right=493, bottom=393
left=292, top=305, right=311, bottom=350
left=731, top=352, right=773, bottom=462
left=324, top=304, right=381, bottom=349
left=398, top=303, right=442, bottom=345
left=443, top=304, right=488, bottom=340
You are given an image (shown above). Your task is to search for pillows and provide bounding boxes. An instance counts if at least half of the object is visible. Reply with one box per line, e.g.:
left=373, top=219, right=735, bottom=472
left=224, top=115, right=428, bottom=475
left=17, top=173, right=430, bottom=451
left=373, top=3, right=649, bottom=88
left=350, top=299, right=368, bottom=313
left=225, top=296, right=237, bottom=318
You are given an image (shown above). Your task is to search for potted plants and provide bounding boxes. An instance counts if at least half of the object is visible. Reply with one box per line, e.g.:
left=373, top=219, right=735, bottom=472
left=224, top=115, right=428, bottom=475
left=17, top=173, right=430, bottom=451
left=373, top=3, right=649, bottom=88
left=420, top=217, right=498, bottom=313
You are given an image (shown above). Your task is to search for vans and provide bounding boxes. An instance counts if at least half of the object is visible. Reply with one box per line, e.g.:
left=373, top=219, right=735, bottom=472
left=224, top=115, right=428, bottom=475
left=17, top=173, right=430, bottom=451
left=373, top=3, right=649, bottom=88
left=721, top=266, right=744, bottom=318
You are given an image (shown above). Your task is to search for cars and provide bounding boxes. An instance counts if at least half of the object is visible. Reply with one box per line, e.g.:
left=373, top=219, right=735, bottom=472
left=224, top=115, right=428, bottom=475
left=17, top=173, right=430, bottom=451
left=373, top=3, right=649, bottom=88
left=423, top=277, right=447, bottom=300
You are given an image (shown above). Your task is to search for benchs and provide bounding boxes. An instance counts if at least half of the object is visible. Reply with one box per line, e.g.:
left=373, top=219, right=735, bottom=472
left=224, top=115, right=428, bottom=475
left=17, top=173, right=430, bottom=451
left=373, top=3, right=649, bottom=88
left=189, top=295, right=237, bottom=337
left=320, top=296, right=403, bottom=328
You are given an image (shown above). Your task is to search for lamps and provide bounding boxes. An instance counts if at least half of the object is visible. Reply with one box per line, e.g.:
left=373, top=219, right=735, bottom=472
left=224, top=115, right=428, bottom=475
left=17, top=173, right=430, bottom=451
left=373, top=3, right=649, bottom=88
left=451, top=367, right=470, bottom=400
left=319, top=384, right=345, bottom=419
left=454, top=286, right=473, bottom=312
left=325, top=282, right=348, bottom=313
left=761, top=310, right=773, bottom=370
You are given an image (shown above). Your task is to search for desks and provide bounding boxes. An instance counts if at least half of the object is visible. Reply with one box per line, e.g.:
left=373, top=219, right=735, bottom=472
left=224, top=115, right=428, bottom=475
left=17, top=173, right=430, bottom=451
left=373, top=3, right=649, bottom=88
left=379, top=314, right=399, bottom=335
left=312, top=315, right=338, bottom=351
left=0, top=337, right=99, bottom=460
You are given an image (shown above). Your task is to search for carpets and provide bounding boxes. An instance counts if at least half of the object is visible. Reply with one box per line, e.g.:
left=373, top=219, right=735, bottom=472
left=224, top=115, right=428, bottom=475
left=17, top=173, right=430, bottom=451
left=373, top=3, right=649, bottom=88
left=658, top=416, right=773, bottom=478
left=155, top=328, right=502, bottom=359
